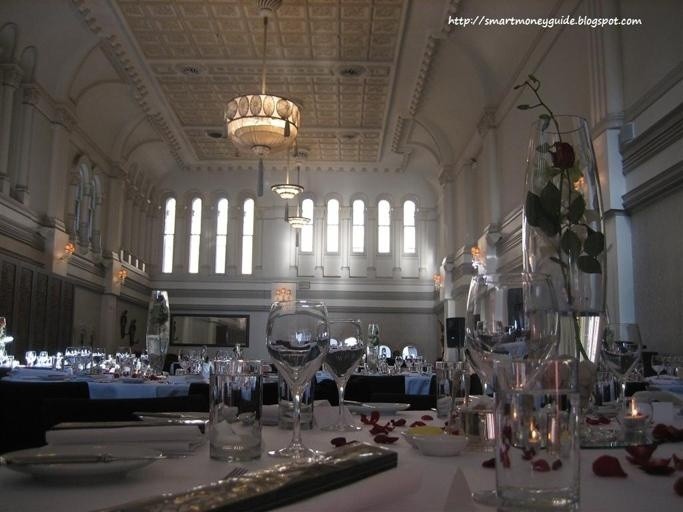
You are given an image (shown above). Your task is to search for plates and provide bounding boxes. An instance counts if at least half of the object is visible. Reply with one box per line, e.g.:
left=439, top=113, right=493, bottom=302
left=123, top=377, right=145, bottom=384
left=0, top=444, right=162, bottom=482
left=346, top=402, right=411, bottom=415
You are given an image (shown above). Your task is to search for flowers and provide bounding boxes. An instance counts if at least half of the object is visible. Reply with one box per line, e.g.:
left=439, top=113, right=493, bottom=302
left=519, top=78, right=602, bottom=367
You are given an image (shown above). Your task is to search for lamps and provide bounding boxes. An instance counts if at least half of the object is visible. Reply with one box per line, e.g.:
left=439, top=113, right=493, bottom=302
left=270, top=158, right=312, bottom=231
left=56, top=243, right=74, bottom=262
left=468, top=246, right=484, bottom=267
left=225, top=0, right=302, bottom=158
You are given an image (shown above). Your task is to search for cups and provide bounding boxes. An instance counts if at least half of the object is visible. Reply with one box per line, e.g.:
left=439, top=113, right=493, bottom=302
left=435, top=360, right=471, bottom=417
left=492, top=356, right=581, bottom=510
left=378, top=355, right=388, bottom=374
left=208, top=360, right=264, bottom=461
left=190, top=352, right=202, bottom=375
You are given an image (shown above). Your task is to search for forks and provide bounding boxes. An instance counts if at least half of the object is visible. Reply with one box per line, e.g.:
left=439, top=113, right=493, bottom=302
left=223, top=466, right=248, bottom=480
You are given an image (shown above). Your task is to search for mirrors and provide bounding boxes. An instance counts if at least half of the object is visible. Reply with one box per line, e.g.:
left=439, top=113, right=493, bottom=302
left=169, top=313, right=251, bottom=349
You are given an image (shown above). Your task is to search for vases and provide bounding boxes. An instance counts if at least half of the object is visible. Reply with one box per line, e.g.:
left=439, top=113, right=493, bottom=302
left=524, top=111, right=605, bottom=410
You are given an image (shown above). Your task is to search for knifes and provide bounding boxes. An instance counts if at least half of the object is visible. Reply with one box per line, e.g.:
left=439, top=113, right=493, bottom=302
left=7, top=454, right=169, bottom=465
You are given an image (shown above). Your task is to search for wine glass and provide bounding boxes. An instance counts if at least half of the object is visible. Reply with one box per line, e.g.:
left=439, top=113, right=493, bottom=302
left=395, top=355, right=422, bottom=374
left=178, top=350, right=191, bottom=374
left=65, top=344, right=131, bottom=376
left=265, top=300, right=329, bottom=459
left=26, top=351, right=48, bottom=367
left=651, top=353, right=683, bottom=383
left=321, top=320, right=363, bottom=432
left=599, top=323, right=642, bottom=411
left=466, top=271, right=559, bottom=508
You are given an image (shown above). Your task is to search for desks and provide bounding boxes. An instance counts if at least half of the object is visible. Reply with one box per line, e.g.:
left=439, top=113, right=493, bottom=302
left=2, top=348, right=681, bottom=512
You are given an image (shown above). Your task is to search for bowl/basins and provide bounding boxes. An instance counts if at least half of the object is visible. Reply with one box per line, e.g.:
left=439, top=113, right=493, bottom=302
left=401, top=429, right=466, bottom=457
